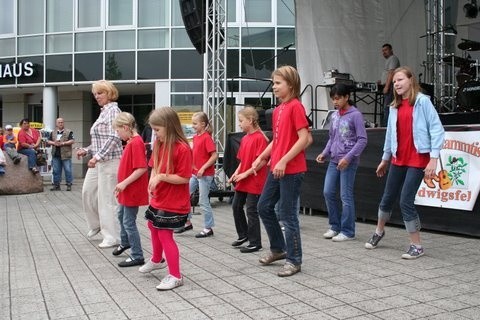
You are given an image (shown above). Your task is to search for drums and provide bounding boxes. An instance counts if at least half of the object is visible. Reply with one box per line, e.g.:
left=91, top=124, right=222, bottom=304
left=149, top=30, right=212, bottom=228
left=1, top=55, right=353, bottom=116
left=456, top=81, right=480, bottom=113
left=456, top=60, right=480, bottom=87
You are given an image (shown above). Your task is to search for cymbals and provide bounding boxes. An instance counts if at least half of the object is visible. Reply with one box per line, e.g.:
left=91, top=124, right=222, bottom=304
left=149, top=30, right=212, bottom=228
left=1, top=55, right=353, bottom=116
left=458, top=41, right=480, bottom=51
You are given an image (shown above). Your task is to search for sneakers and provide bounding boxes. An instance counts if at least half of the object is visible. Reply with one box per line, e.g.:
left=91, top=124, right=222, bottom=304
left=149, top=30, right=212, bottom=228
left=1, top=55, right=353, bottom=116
left=401, top=245, right=424, bottom=259
left=365, top=229, right=385, bottom=248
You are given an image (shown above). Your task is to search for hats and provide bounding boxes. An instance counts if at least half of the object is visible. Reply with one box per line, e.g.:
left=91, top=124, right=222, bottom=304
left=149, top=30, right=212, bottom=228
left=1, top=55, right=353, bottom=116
left=4, top=125, right=13, bottom=130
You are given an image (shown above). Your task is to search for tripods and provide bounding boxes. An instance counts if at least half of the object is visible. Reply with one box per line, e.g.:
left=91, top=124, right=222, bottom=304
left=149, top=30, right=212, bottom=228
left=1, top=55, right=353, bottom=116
left=436, top=53, right=465, bottom=113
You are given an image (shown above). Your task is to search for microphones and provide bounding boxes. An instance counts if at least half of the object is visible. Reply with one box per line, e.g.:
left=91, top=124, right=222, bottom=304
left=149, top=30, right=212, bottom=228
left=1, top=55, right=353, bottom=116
left=284, top=42, right=295, bottom=50
left=450, top=24, right=458, bottom=34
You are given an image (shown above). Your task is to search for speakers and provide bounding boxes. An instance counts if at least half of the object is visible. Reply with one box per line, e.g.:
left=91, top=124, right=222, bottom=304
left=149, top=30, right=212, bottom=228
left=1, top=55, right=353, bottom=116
left=266, top=109, right=313, bottom=130
left=179, top=0, right=225, bottom=54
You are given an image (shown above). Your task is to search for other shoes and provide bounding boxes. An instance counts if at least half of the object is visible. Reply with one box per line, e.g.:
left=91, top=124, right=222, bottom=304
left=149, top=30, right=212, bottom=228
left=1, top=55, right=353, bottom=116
left=195, top=229, right=214, bottom=238
left=66, top=183, right=72, bottom=190
left=139, top=257, right=167, bottom=273
left=240, top=244, right=259, bottom=252
left=173, top=222, right=193, bottom=233
left=14, top=157, right=21, bottom=164
left=119, top=257, right=144, bottom=267
left=278, top=259, right=301, bottom=276
left=332, top=232, right=355, bottom=242
left=259, top=251, right=287, bottom=263
left=323, top=229, right=338, bottom=238
left=50, top=184, right=61, bottom=191
left=156, top=274, right=183, bottom=291
left=32, top=167, right=39, bottom=174
left=99, top=240, right=121, bottom=248
left=87, top=227, right=100, bottom=238
left=231, top=236, right=248, bottom=246
left=112, top=246, right=131, bottom=255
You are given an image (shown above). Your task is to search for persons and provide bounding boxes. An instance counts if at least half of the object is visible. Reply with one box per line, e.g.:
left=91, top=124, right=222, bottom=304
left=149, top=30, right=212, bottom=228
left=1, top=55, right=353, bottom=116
left=76, top=80, right=123, bottom=248
left=251, top=65, right=314, bottom=277
left=46, top=118, right=76, bottom=191
left=315, top=84, right=368, bottom=242
left=173, top=112, right=217, bottom=238
left=138, top=106, right=192, bottom=290
left=112, top=112, right=150, bottom=267
left=228, top=106, right=269, bottom=253
left=376, top=43, right=400, bottom=126
left=17, top=119, right=41, bottom=174
left=365, top=66, right=446, bottom=259
left=0, top=124, right=21, bottom=165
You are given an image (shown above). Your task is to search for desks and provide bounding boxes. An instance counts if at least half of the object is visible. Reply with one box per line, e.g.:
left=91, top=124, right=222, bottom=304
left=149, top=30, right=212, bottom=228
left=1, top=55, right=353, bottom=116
left=352, top=85, right=386, bottom=127
